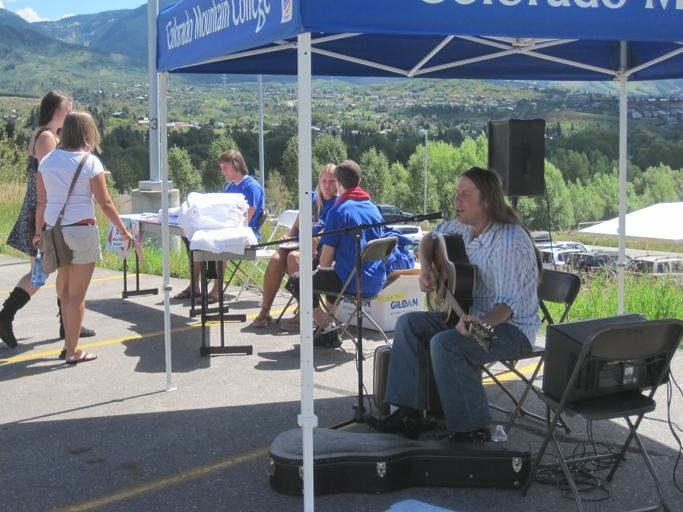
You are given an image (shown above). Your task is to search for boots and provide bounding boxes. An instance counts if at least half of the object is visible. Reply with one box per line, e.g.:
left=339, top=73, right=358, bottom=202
left=57, top=298, right=96, bottom=339
left=0, top=287, right=30, bottom=347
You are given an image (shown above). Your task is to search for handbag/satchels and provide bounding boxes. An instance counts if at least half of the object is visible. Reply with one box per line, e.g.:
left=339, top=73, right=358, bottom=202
left=39, top=225, right=72, bottom=273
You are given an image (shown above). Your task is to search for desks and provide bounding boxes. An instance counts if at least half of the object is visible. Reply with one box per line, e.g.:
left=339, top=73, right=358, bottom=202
left=105, top=213, right=229, bottom=318
left=191, top=228, right=259, bottom=356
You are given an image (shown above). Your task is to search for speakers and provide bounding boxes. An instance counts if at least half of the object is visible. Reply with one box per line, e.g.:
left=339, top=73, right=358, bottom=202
left=543, top=315, right=668, bottom=402
left=488, top=118, right=547, bottom=197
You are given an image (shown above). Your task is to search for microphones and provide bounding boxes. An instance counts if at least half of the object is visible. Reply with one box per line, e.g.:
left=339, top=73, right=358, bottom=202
left=416, top=211, right=452, bottom=221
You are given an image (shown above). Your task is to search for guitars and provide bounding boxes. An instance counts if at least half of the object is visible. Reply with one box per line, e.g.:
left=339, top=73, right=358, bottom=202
left=426, top=233, right=498, bottom=352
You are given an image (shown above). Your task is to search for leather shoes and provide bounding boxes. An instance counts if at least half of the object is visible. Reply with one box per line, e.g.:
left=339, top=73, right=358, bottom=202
left=366, top=407, right=426, bottom=434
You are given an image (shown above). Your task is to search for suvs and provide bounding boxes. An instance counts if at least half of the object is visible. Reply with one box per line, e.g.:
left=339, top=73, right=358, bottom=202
left=385, top=225, right=424, bottom=239
left=528, top=230, right=683, bottom=285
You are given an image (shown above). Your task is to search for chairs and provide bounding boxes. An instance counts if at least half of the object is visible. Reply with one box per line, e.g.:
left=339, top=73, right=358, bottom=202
left=481, top=269, right=581, bottom=436
left=521, top=318, right=683, bottom=511
left=223, top=209, right=398, bottom=360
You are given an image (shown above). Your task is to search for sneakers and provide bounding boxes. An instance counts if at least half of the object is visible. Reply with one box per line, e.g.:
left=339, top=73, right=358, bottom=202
left=173, top=290, right=343, bottom=348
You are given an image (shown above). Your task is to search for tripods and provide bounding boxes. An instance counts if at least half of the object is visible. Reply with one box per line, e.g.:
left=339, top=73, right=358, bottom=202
left=328, top=237, right=384, bottom=428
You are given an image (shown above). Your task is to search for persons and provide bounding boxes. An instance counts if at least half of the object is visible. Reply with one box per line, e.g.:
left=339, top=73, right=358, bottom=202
left=253, top=163, right=340, bottom=328
left=0, top=90, right=96, bottom=348
left=173, top=149, right=264, bottom=305
left=32, top=111, right=134, bottom=363
left=288, top=160, right=387, bottom=347
left=367, top=166, right=541, bottom=443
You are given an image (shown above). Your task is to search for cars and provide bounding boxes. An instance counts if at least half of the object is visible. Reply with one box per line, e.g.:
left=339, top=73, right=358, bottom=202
left=376, top=204, right=414, bottom=222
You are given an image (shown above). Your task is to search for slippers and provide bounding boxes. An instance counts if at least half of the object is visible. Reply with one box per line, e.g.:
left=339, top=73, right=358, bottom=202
left=66, top=351, right=97, bottom=364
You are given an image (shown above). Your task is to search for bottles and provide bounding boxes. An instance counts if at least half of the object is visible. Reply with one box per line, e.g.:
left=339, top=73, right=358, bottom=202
left=491, top=424, right=506, bottom=442
left=31, top=244, right=45, bottom=285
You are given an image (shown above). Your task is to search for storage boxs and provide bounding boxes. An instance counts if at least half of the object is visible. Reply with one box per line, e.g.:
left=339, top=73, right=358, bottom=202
left=335, top=275, right=428, bottom=332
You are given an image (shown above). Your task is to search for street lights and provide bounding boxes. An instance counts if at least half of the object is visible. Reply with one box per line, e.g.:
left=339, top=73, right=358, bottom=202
left=419, top=129, right=428, bottom=215
